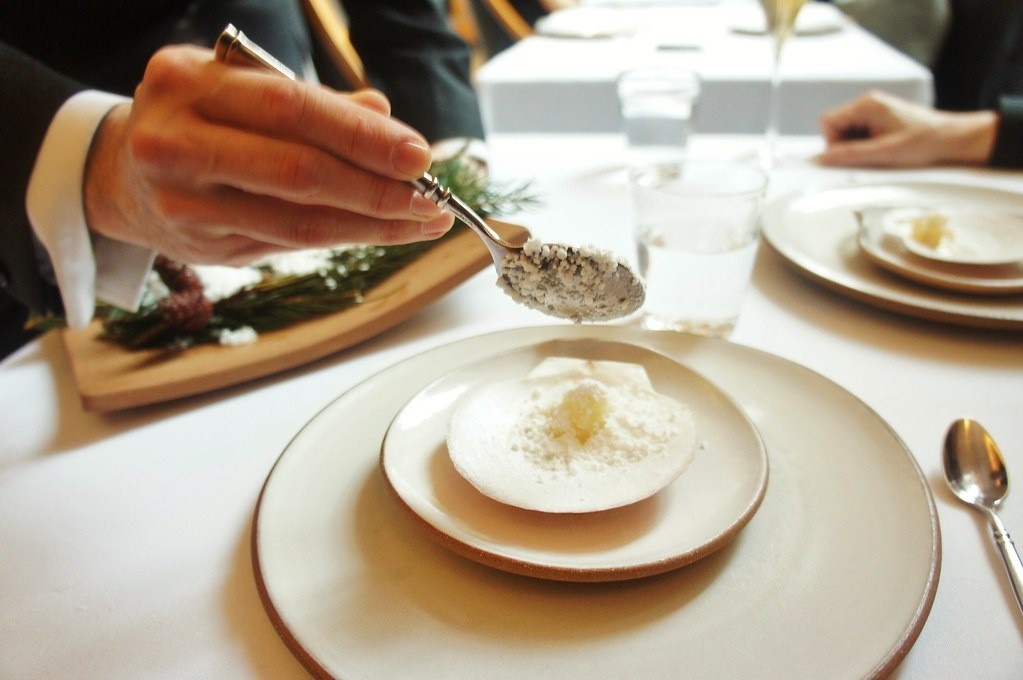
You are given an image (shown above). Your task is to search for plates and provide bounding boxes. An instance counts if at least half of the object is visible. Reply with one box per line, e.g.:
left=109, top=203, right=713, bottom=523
left=762, top=165, right=1023, bottom=328
left=859, top=208, right=1023, bottom=294
left=250, top=324, right=942, bottom=680
left=382, top=338, right=769, bottom=582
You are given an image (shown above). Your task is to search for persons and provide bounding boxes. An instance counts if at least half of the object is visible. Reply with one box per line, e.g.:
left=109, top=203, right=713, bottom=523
left=0, top=0, right=492, bottom=354
left=814, top=0, right=1023, bottom=169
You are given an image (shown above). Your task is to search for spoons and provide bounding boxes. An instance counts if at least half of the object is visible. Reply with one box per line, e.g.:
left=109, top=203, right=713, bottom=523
left=940, top=419, right=1023, bottom=615
left=215, top=24, right=646, bottom=322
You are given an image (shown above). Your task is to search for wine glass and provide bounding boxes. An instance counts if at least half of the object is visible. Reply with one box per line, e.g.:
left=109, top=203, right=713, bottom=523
left=743, top=0, right=806, bottom=163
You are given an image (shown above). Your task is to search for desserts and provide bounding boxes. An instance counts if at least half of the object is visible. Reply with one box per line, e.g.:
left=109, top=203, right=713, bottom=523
left=910, top=213, right=947, bottom=248
left=508, top=381, right=669, bottom=468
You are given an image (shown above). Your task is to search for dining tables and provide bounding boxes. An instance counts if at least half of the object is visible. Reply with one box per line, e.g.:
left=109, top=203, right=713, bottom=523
left=475, top=0, right=935, bottom=134
left=0, top=135, right=1023, bottom=680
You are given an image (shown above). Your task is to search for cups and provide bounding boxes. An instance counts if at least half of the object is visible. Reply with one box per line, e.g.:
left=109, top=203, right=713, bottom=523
left=617, top=66, right=700, bottom=146
left=631, top=157, right=767, bottom=330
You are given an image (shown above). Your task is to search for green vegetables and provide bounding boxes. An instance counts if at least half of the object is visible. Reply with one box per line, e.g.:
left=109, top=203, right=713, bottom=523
left=94, top=149, right=544, bottom=354
